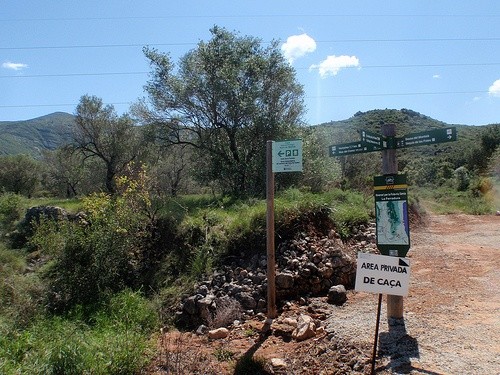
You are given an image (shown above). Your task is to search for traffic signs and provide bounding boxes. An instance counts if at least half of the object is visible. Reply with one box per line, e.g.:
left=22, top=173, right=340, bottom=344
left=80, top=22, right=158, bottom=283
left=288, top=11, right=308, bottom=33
left=329, top=123, right=457, bottom=318
left=271, top=140, right=302, bottom=172
